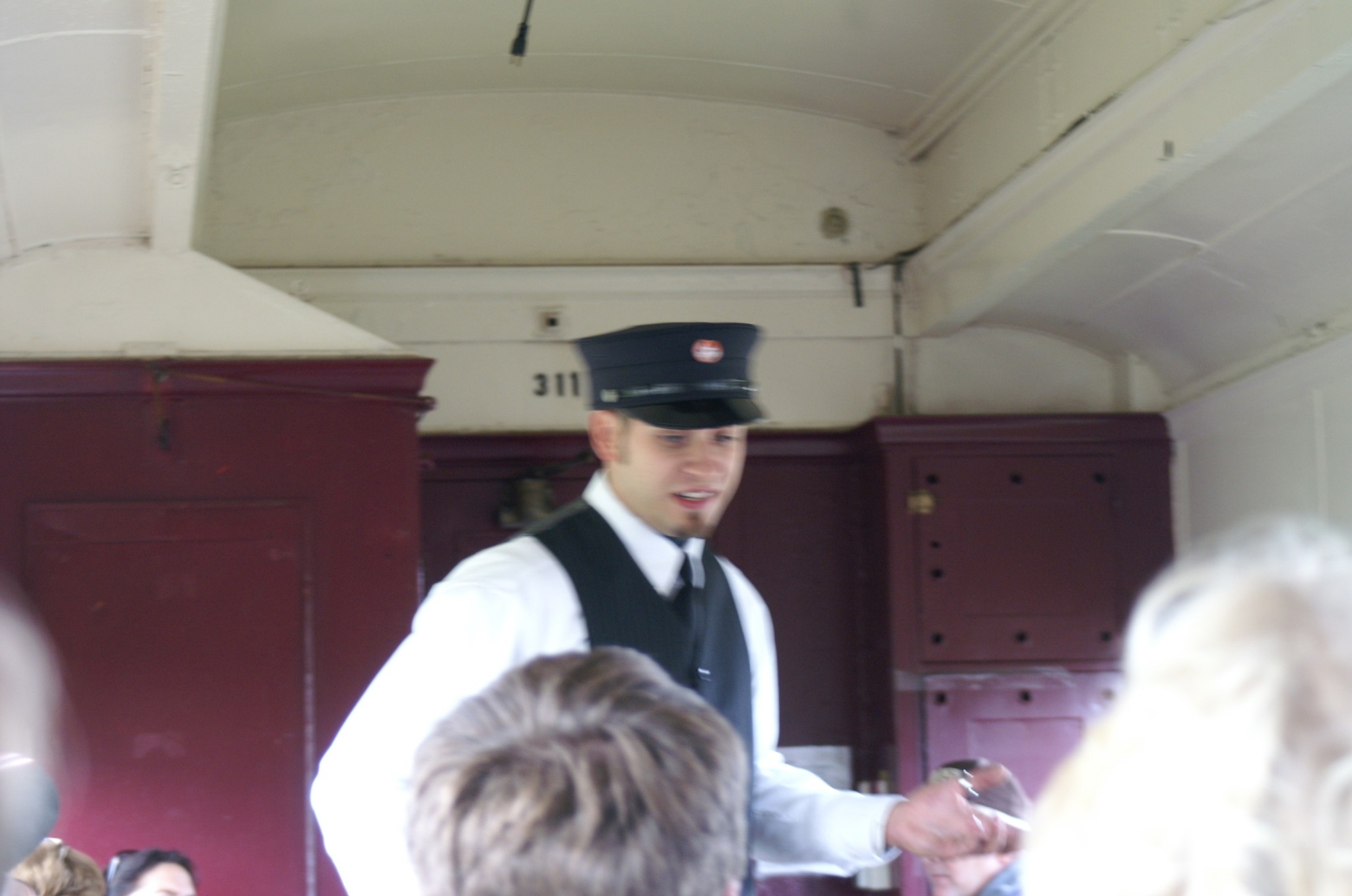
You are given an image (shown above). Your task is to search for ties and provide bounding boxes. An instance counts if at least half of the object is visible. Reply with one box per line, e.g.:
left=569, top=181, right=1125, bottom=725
left=671, top=553, right=701, bottom=663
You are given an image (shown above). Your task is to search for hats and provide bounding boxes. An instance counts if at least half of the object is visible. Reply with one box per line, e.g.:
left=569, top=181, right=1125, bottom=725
left=573, top=321, right=763, bottom=430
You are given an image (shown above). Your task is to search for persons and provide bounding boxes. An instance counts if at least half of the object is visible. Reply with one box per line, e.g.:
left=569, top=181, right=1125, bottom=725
left=0, top=838, right=199, bottom=896
left=1021, top=517, right=1351, bottom=896
left=923, top=757, right=1033, bottom=896
left=307, top=322, right=1031, bottom=896
left=406, top=647, right=748, bottom=896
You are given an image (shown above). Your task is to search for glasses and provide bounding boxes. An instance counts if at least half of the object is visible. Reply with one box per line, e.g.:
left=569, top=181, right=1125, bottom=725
left=107, top=850, right=138, bottom=882
left=44, top=837, right=68, bottom=862
